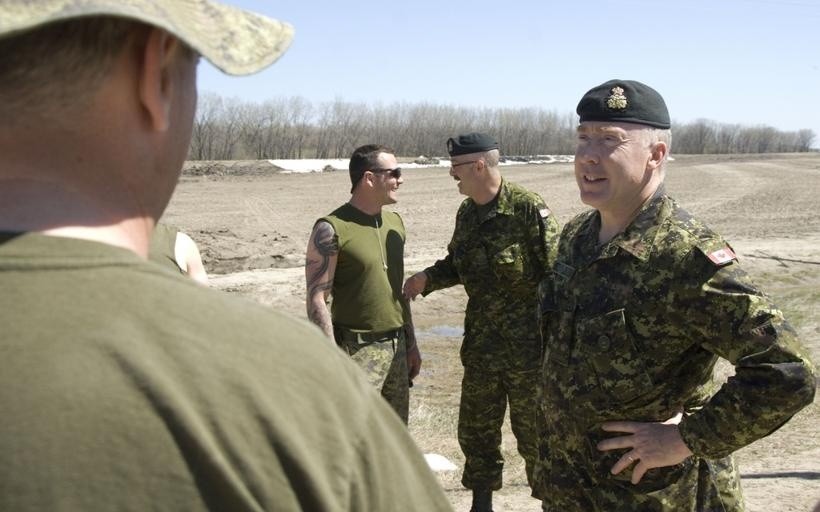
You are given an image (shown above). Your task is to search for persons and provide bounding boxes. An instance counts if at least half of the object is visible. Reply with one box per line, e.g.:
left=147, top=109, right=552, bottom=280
left=526, top=79, right=818, bottom=512
left=398, top=128, right=565, bottom=512
left=302, top=141, right=424, bottom=429
left=147, top=218, right=210, bottom=288
left=1, top=2, right=457, bottom=512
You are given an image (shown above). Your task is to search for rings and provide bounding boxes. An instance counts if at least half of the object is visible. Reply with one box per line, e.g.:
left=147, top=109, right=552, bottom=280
left=625, top=452, right=634, bottom=461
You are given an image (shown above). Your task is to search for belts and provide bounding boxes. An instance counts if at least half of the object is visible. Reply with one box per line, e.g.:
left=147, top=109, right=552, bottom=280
left=334, top=326, right=405, bottom=345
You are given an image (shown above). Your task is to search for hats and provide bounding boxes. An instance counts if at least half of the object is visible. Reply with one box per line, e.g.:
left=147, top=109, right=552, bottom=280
left=575, top=79, right=670, bottom=130
left=1, top=0, right=295, bottom=77
left=445, top=130, right=501, bottom=157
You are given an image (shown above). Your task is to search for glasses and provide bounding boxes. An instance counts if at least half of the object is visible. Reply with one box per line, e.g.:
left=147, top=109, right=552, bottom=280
left=450, top=162, right=479, bottom=169
left=367, top=166, right=401, bottom=180
